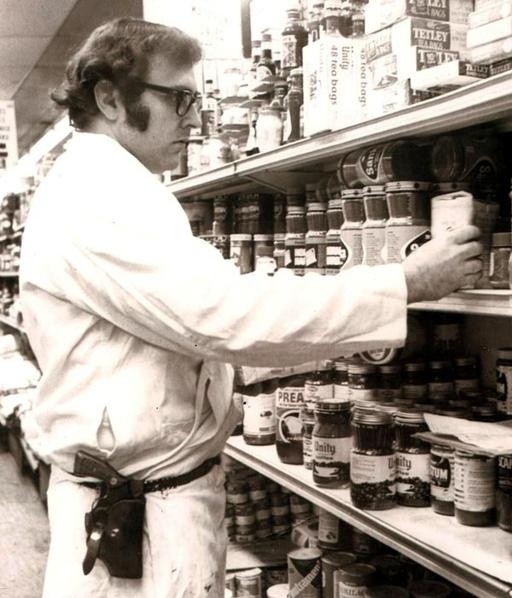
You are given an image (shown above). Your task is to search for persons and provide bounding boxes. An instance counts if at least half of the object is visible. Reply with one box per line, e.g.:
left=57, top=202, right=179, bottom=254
left=19, top=17, right=485, bottom=598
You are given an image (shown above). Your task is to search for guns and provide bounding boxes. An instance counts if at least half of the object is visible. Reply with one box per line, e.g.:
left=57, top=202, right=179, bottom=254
left=74, top=450, right=146, bottom=528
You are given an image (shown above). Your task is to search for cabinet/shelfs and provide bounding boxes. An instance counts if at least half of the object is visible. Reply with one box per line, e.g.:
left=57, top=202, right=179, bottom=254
left=0, top=227, right=28, bottom=334
left=163, top=66, right=512, bottom=598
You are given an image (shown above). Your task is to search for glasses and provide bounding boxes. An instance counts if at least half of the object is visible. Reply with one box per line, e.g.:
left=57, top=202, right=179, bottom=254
left=142, top=82, right=202, bottom=117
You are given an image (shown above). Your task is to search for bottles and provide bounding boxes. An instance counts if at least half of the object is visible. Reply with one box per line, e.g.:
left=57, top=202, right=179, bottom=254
left=165, top=0, right=369, bottom=182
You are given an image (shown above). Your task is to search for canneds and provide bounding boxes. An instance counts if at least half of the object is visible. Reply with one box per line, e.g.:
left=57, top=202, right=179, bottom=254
left=181, top=135, right=512, bottom=290
left=224, top=566, right=289, bottom=598
left=313, top=398, right=512, bottom=531
left=495, top=347, right=512, bottom=420
left=229, top=314, right=483, bottom=469
left=287, top=507, right=476, bottom=598
left=222, top=454, right=319, bottom=548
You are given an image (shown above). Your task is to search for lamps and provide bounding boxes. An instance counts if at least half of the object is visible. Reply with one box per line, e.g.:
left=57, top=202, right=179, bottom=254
left=18, top=106, right=77, bottom=167
